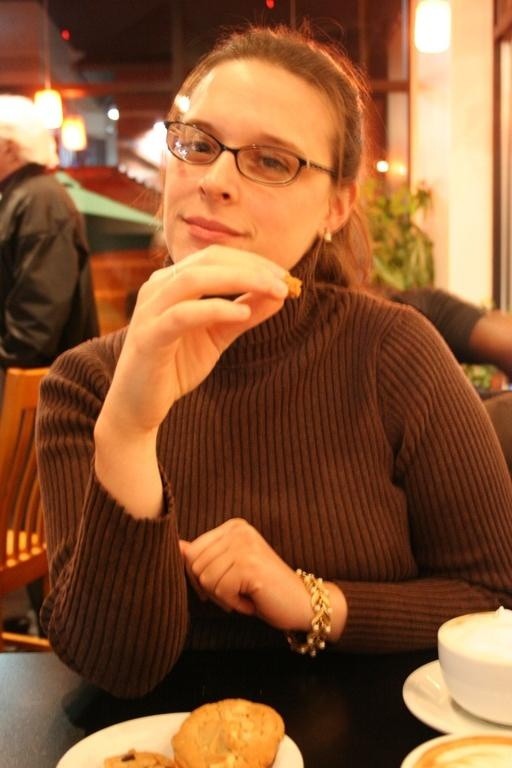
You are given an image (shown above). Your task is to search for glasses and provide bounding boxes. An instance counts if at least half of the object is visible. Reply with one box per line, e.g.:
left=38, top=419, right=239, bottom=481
left=163, top=119, right=337, bottom=186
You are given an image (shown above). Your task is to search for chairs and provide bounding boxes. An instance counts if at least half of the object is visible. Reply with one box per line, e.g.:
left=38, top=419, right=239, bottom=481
left=2, top=364, right=52, bottom=655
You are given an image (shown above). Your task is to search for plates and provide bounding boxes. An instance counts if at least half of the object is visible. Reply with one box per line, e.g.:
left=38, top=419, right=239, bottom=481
left=402, top=655, right=512, bottom=735
left=52, top=710, right=304, bottom=768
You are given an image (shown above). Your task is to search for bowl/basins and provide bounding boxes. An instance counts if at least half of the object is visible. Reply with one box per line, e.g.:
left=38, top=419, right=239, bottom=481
left=438, top=606, right=511, bottom=728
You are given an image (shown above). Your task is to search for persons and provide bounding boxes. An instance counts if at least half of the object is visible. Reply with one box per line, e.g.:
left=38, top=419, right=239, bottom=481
left=390, top=283, right=510, bottom=386
left=40, top=21, right=511, bottom=699
left=0, top=91, right=102, bottom=375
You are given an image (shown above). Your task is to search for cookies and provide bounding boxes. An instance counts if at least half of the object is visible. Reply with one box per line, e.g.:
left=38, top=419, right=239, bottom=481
left=102, top=752, right=180, bottom=768
left=171, top=697, right=286, bottom=768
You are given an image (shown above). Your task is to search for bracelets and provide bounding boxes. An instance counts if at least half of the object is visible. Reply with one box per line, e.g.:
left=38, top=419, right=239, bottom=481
left=281, top=569, right=334, bottom=659
